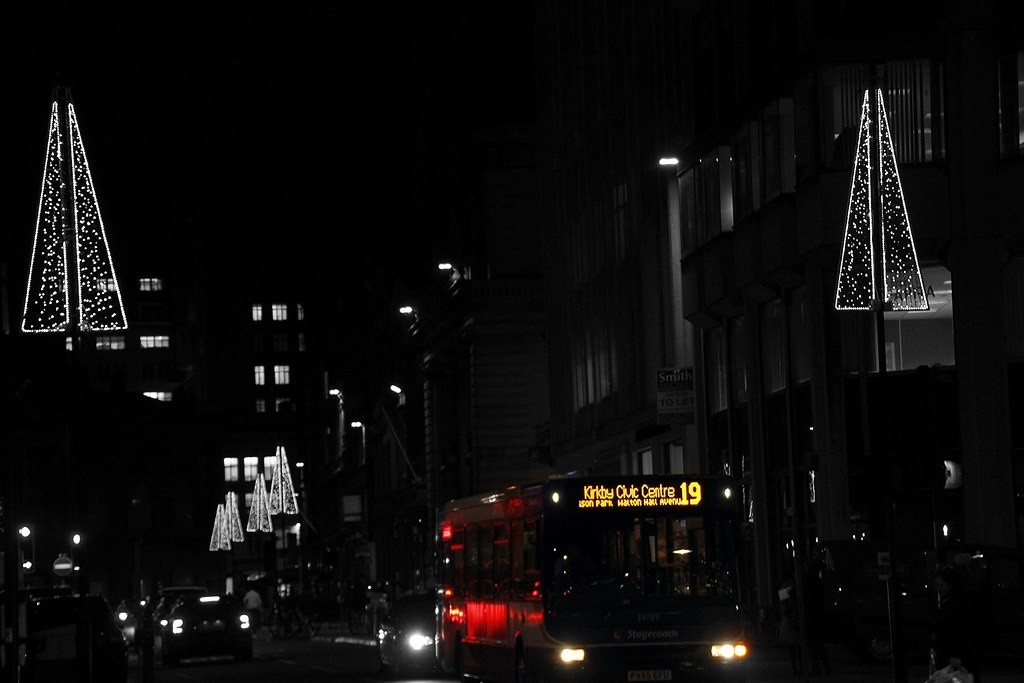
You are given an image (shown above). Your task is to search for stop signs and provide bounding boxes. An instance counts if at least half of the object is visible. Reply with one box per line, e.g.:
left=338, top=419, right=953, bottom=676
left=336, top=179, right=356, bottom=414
left=54, top=556, right=74, bottom=576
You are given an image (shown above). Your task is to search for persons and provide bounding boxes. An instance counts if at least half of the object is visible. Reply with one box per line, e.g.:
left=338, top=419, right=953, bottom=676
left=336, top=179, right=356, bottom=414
left=788, top=587, right=834, bottom=677
left=934, top=569, right=983, bottom=683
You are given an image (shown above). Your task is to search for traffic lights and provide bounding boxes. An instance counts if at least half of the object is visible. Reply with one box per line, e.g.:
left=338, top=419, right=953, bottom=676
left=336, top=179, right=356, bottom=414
left=17, top=523, right=34, bottom=572
left=69, top=530, right=87, bottom=577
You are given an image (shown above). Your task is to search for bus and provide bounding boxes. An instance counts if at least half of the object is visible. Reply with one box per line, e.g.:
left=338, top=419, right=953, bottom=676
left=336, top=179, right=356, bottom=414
left=438, top=471, right=751, bottom=683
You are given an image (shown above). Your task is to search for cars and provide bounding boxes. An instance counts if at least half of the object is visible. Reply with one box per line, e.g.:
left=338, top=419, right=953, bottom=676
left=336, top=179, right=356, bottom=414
left=149, top=588, right=253, bottom=667
left=375, top=595, right=457, bottom=680
left=24, top=595, right=129, bottom=679
